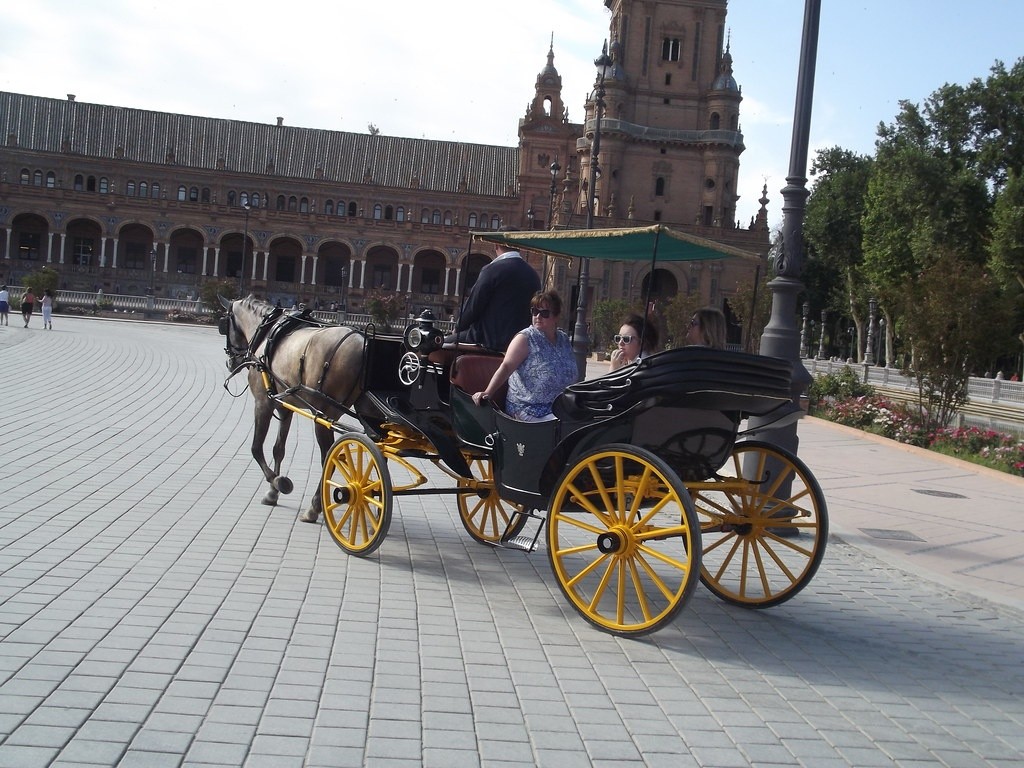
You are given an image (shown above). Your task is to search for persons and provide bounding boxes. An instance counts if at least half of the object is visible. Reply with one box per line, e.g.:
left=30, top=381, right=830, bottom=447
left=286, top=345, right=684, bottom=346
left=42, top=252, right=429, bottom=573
left=438, top=225, right=542, bottom=404
left=607, top=312, right=659, bottom=374
left=684, top=308, right=727, bottom=350
left=19, top=287, right=53, bottom=330
left=263, top=298, right=342, bottom=311
left=1010, top=372, right=1020, bottom=382
left=472, top=288, right=579, bottom=423
left=665, top=339, right=674, bottom=350
left=409, top=310, right=415, bottom=326
left=0, top=285, right=9, bottom=326
left=984, top=370, right=992, bottom=378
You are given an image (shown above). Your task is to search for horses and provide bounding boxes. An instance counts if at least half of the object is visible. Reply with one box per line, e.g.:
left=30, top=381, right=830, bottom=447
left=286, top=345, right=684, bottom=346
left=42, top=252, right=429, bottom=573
left=217, top=291, right=388, bottom=535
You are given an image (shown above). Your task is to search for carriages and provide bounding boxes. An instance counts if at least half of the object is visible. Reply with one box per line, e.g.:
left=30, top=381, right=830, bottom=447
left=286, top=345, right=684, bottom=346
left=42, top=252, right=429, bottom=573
left=216, top=290, right=828, bottom=639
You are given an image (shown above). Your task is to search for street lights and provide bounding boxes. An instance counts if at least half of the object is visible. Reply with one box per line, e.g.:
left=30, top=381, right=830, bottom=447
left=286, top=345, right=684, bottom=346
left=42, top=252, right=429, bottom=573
left=873, top=318, right=885, bottom=367
left=570, top=37, right=615, bottom=381
left=238, top=198, right=252, bottom=297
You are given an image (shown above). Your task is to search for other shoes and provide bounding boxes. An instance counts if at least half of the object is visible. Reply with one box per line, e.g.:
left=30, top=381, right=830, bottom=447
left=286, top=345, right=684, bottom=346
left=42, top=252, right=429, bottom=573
left=50, top=325, right=51, bottom=329
left=24, top=325, right=28, bottom=328
left=44, top=327, right=47, bottom=328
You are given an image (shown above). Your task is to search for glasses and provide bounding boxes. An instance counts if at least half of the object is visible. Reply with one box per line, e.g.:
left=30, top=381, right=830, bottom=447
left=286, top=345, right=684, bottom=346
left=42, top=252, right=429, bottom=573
left=690, top=319, right=699, bottom=327
left=614, top=335, right=638, bottom=345
left=531, top=307, right=552, bottom=319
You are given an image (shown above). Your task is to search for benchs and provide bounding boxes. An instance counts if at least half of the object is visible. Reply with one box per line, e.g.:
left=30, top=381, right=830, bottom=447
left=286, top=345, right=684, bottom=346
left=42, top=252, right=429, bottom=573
left=445, top=346, right=817, bottom=512
